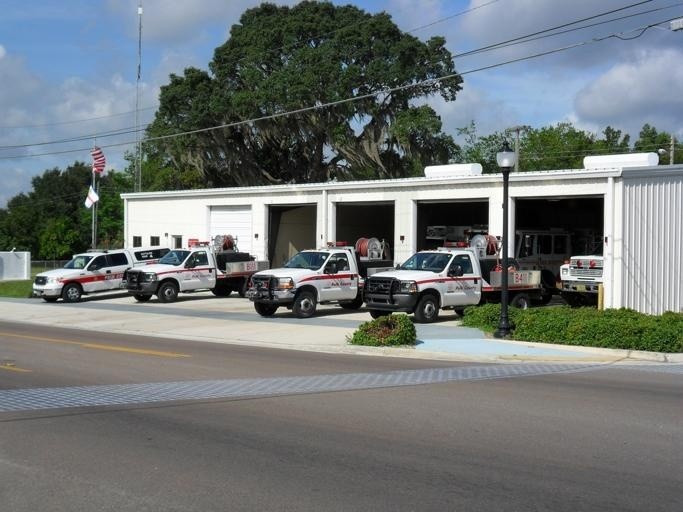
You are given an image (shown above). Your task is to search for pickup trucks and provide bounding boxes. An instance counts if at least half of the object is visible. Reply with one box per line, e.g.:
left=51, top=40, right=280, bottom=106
left=31, top=245, right=180, bottom=303
left=463, top=225, right=572, bottom=305
left=244, top=237, right=401, bottom=318
left=118, top=235, right=258, bottom=303
left=555, top=231, right=603, bottom=309
left=423, top=225, right=471, bottom=250
left=359, top=234, right=542, bottom=324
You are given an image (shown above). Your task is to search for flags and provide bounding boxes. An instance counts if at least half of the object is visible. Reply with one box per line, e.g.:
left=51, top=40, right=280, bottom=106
left=91, top=147, right=105, bottom=173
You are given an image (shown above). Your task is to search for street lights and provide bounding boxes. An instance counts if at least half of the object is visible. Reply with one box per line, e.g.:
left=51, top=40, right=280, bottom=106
left=657, top=148, right=674, bottom=165
left=493, top=137, right=516, bottom=339
